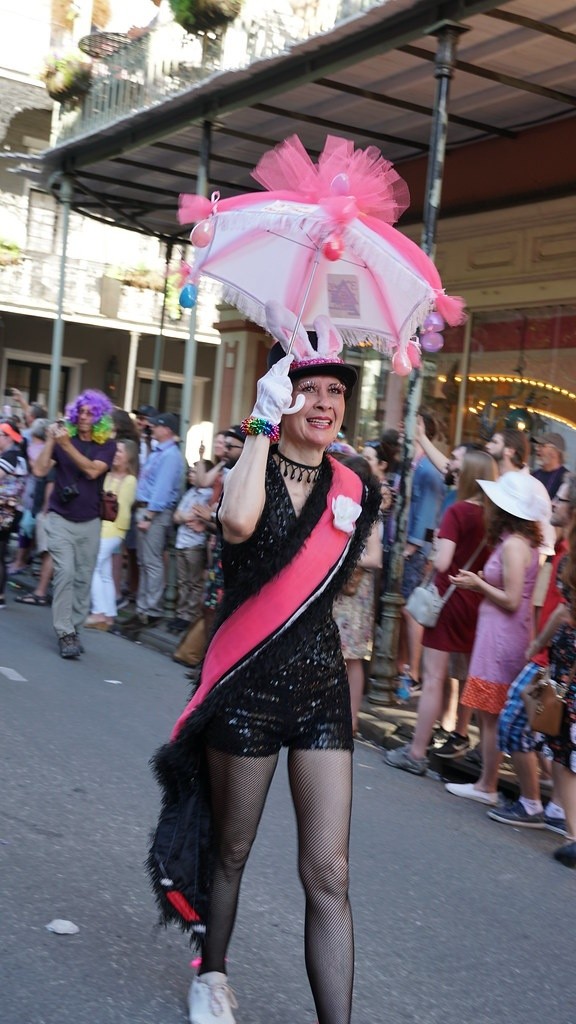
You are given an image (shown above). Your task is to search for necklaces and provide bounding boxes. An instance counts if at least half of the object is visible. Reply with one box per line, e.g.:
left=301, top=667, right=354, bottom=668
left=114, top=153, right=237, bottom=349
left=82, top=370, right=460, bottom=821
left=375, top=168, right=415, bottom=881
left=273, top=448, right=321, bottom=482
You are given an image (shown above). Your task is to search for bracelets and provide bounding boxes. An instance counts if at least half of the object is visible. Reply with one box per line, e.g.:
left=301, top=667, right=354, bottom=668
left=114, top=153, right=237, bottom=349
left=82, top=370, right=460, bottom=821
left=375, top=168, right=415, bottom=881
left=144, top=516, right=151, bottom=521
left=243, top=418, right=280, bottom=442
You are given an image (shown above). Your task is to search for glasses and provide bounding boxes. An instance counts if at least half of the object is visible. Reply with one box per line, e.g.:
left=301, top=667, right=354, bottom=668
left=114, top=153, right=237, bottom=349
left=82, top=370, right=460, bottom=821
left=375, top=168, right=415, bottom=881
left=554, top=494, right=571, bottom=505
left=225, top=443, right=241, bottom=450
left=364, top=441, right=385, bottom=454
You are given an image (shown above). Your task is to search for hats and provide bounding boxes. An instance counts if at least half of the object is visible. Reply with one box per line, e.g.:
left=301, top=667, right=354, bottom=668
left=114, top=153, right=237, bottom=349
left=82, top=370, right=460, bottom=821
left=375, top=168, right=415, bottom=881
left=225, top=423, right=246, bottom=443
left=147, top=412, right=179, bottom=430
left=131, top=404, right=157, bottom=419
left=530, top=432, right=565, bottom=452
left=264, top=296, right=358, bottom=394
left=474, top=470, right=550, bottom=523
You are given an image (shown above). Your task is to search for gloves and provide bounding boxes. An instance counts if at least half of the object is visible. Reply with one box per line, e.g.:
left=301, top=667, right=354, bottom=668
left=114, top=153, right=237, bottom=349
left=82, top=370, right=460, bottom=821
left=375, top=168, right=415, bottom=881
left=248, top=354, right=293, bottom=426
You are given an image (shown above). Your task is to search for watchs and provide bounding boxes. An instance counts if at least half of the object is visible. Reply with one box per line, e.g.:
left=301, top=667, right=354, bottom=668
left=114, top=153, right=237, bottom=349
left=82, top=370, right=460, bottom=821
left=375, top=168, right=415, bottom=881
left=210, top=511, right=216, bottom=523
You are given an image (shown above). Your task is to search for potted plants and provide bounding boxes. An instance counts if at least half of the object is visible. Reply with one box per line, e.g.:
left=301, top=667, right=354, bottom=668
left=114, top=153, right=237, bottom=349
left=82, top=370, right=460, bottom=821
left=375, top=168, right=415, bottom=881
left=169, top=0, right=240, bottom=34
left=40, top=57, right=91, bottom=102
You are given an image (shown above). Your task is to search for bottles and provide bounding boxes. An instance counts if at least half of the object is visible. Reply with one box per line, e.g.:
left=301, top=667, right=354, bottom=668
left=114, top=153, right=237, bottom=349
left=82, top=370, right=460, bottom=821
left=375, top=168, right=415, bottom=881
left=394, top=664, right=411, bottom=706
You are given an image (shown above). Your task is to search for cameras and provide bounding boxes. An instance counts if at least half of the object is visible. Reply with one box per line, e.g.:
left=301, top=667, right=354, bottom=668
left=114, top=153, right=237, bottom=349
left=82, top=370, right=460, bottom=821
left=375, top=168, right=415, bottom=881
left=60, top=484, right=79, bottom=504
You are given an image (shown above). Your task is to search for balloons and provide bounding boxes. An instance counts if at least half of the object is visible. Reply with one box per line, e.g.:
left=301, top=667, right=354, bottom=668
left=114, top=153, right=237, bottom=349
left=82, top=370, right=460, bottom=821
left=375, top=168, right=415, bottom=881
left=191, top=219, right=213, bottom=248
left=324, top=232, right=343, bottom=260
left=422, top=312, right=444, bottom=350
left=393, top=342, right=422, bottom=374
left=180, top=285, right=197, bottom=307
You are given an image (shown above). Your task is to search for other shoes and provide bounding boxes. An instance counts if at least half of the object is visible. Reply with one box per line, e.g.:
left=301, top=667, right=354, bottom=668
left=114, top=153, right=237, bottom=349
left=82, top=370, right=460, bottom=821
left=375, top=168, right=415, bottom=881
left=0, top=562, right=193, bottom=657
left=443, top=782, right=498, bottom=806
left=486, top=799, right=546, bottom=829
left=543, top=804, right=568, bottom=836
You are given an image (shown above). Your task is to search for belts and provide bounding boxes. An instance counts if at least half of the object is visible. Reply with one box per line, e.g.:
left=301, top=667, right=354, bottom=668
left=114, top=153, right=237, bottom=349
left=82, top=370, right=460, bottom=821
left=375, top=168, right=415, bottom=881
left=134, top=501, right=148, bottom=507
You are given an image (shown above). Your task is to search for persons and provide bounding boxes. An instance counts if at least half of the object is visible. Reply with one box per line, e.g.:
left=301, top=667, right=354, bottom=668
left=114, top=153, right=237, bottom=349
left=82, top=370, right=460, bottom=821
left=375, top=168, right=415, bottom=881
left=333, top=416, right=575, bottom=867
left=1, top=387, right=248, bottom=667
left=33, top=390, right=116, bottom=658
left=445, top=471, right=556, bottom=807
left=146, top=298, right=383, bottom=1023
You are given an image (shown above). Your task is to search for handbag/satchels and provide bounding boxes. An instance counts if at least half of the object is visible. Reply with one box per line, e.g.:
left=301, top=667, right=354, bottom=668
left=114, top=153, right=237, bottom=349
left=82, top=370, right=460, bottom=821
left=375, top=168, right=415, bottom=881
left=518, top=665, right=569, bottom=738
left=401, top=585, right=445, bottom=629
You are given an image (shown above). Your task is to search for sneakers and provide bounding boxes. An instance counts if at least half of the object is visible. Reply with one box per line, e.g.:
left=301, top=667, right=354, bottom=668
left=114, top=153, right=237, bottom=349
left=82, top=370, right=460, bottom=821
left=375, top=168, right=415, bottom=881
left=434, top=734, right=471, bottom=759
left=384, top=747, right=426, bottom=775
left=186, top=970, right=239, bottom=1024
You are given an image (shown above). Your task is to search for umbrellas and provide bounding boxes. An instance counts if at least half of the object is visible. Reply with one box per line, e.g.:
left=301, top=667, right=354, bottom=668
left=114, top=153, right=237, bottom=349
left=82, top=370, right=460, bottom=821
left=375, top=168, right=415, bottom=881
left=177, top=133, right=465, bottom=414
